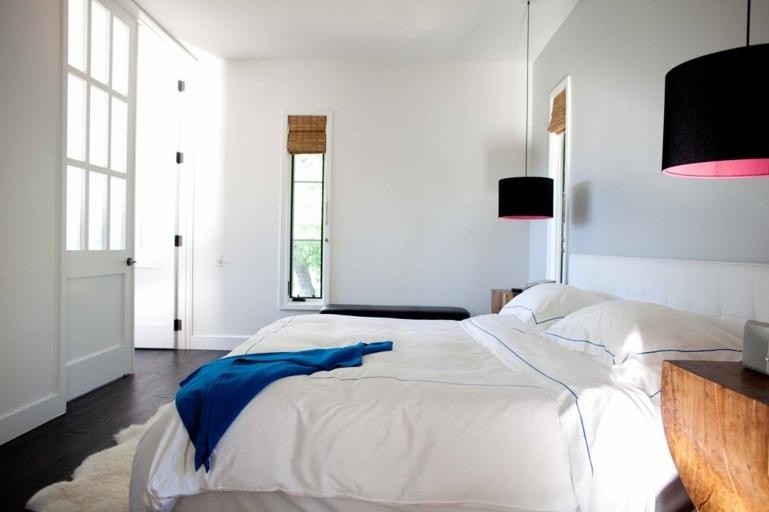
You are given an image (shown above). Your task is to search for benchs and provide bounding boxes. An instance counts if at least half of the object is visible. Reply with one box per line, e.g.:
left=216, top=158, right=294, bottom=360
left=321, top=303, right=470, bottom=320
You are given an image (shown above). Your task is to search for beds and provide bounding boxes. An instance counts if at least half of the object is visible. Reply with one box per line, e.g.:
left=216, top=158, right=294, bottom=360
left=132, top=255, right=769, bottom=512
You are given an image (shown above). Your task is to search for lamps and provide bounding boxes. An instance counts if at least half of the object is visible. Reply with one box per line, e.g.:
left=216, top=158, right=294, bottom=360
left=662, top=0, right=768, bottom=178
left=498, top=2, right=553, bottom=219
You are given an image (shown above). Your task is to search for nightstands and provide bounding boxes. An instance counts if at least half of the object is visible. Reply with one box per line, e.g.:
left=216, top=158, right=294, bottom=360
left=492, top=290, right=524, bottom=316
left=661, top=360, right=768, bottom=512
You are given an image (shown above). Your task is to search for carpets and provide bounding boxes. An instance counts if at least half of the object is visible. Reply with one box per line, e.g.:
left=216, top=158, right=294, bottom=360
left=27, top=403, right=176, bottom=512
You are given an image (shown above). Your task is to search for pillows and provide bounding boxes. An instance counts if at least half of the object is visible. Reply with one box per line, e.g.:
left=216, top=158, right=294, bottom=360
left=545, top=300, right=744, bottom=397
left=498, top=282, right=605, bottom=333
